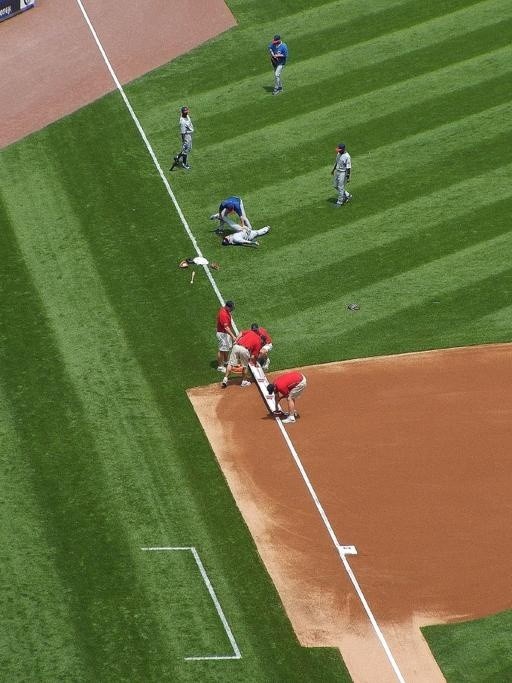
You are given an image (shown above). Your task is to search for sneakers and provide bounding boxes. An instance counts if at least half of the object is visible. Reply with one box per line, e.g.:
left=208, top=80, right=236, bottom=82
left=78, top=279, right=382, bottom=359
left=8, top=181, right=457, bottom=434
left=282, top=409, right=298, bottom=424
left=174, top=155, right=191, bottom=169
left=217, top=366, right=226, bottom=373
left=241, top=380, right=251, bottom=387
left=222, top=376, right=228, bottom=386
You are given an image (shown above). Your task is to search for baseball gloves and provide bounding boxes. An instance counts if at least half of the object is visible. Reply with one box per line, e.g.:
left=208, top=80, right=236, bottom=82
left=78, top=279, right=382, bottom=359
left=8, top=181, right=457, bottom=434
left=210, top=262, right=220, bottom=270
left=348, top=303, right=360, bottom=310
left=179, top=260, right=188, bottom=268
left=271, top=56, right=280, bottom=66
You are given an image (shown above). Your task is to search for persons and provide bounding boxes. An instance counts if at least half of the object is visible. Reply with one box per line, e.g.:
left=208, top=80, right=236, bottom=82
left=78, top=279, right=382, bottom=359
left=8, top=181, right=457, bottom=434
left=268, top=35, right=288, bottom=96
left=330, top=142, right=353, bottom=208
left=174, top=106, right=194, bottom=169
left=210, top=196, right=271, bottom=246
left=216, top=300, right=274, bottom=388
left=266, top=371, right=308, bottom=423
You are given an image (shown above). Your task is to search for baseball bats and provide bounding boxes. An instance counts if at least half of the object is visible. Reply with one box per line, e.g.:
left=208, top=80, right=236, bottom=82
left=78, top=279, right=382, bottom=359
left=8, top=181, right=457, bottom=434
left=169, top=149, right=184, bottom=171
left=190, top=268, right=195, bottom=283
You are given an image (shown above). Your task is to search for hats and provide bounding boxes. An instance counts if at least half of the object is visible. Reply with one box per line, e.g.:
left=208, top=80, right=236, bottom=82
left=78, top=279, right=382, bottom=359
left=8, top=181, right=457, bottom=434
left=251, top=323, right=258, bottom=331
left=336, top=144, right=345, bottom=152
left=226, top=301, right=235, bottom=309
left=267, top=384, right=274, bottom=394
left=273, top=35, right=280, bottom=43
